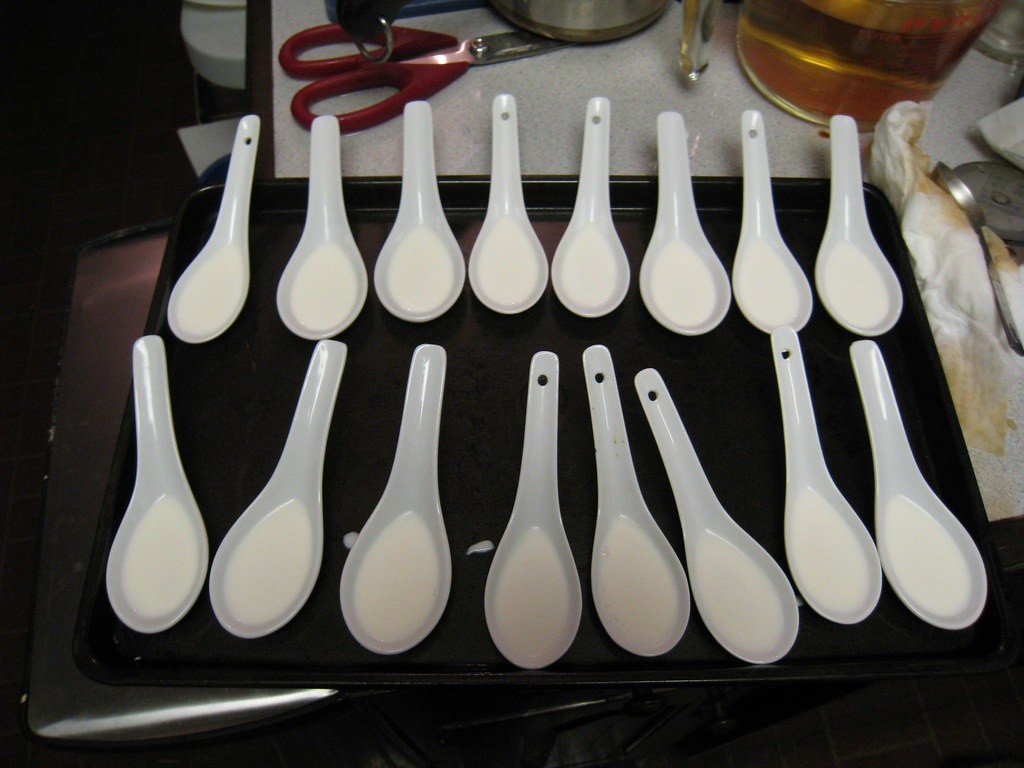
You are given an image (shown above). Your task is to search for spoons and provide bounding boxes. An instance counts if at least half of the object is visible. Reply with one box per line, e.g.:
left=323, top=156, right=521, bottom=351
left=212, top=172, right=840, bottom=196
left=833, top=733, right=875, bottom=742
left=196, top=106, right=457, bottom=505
left=277, top=115, right=369, bottom=340
left=374, top=101, right=465, bottom=323
left=635, top=369, right=799, bottom=664
left=551, top=97, right=631, bottom=318
left=485, top=351, right=582, bottom=670
left=934, top=162, right=1024, bottom=358
left=106, top=335, right=209, bottom=634
left=815, top=116, right=903, bottom=336
left=772, top=327, right=882, bottom=624
left=468, top=93, right=549, bottom=313
left=639, top=112, right=731, bottom=335
left=339, top=344, right=452, bottom=655
left=209, top=340, right=347, bottom=638
left=583, top=345, right=691, bottom=656
left=167, top=115, right=259, bottom=343
left=732, top=111, right=814, bottom=334
left=850, top=341, right=987, bottom=630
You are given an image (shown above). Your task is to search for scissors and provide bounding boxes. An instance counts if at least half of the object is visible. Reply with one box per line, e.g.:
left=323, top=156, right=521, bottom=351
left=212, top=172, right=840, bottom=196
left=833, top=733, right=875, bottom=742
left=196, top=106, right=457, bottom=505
left=278, top=21, right=580, bottom=135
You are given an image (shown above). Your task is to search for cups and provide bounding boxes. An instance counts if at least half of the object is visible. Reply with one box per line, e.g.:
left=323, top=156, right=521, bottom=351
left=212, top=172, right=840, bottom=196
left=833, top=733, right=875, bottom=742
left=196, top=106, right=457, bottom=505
left=736, top=0, right=1003, bottom=129
